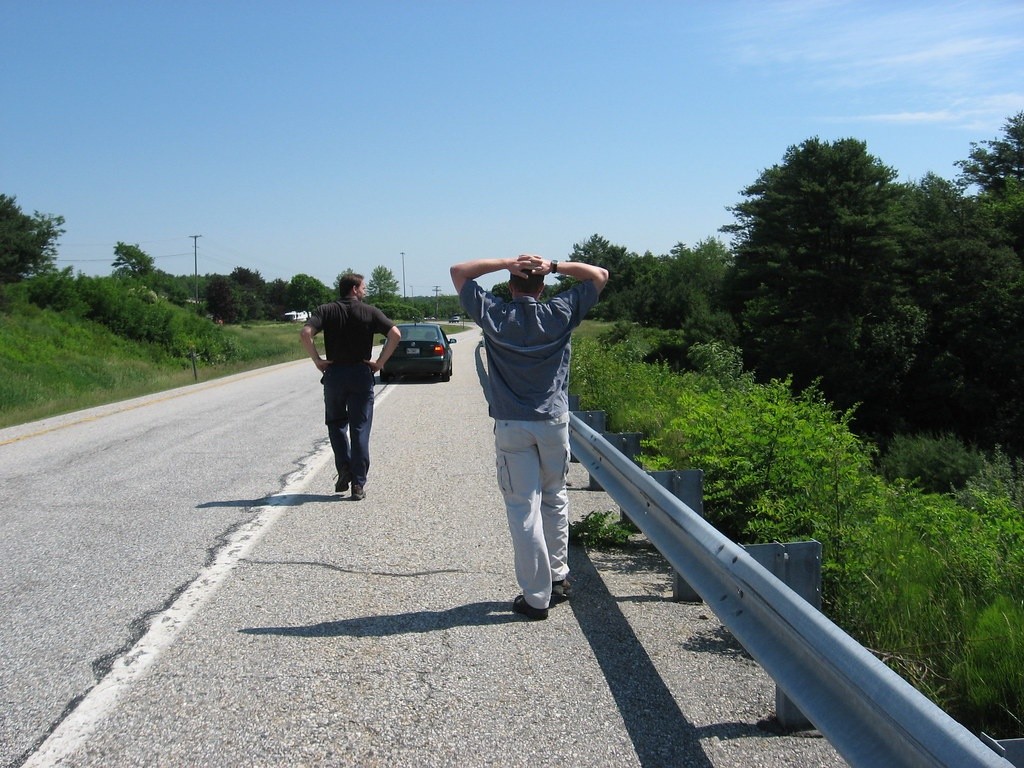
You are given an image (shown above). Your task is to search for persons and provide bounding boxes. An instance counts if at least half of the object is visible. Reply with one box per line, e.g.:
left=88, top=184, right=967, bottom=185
left=219, top=316, right=223, bottom=326
left=450, top=254, right=609, bottom=620
left=300, top=273, right=401, bottom=499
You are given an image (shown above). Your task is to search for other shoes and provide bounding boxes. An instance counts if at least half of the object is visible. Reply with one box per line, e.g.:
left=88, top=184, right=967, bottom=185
left=551, top=579, right=575, bottom=597
left=514, top=594, right=548, bottom=620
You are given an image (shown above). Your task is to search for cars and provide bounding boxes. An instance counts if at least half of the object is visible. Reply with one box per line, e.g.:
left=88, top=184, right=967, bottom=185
left=380, top=324, right=457, bottom=382
left=449, top=317, right=460, bottom=323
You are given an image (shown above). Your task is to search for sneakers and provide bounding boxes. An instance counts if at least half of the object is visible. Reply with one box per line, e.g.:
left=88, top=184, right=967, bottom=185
left=333, top=462, right=352, bottom=492
left=351, top=484, right=367, bottom=500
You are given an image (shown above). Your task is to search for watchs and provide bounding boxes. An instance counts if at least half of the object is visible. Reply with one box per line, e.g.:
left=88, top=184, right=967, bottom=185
left=550, top=259, right=560, bottom=274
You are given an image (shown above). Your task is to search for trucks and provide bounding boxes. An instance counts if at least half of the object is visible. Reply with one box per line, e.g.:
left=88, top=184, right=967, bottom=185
left=285, top=311, right=312, bottom=322
